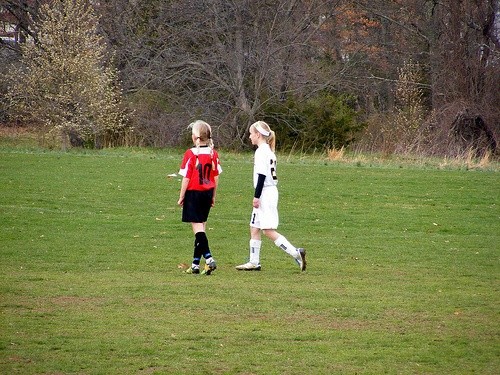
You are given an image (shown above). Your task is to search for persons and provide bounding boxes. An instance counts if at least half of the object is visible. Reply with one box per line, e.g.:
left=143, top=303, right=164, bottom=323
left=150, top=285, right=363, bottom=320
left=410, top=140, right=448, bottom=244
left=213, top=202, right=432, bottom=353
left=234, top=121, right=307, bottom=272
left=176, top=121, right=222, bottom=276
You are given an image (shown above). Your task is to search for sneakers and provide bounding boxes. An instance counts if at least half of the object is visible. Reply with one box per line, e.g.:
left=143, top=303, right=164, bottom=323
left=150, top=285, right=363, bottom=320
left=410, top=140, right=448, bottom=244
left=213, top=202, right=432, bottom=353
left=182, top=267, right=200, bottom=274
left=201, top=261, right=217, bottom=275
left=235, top=263, right=261, bottom=270
left=294, top=248, right=307, bottom=271
